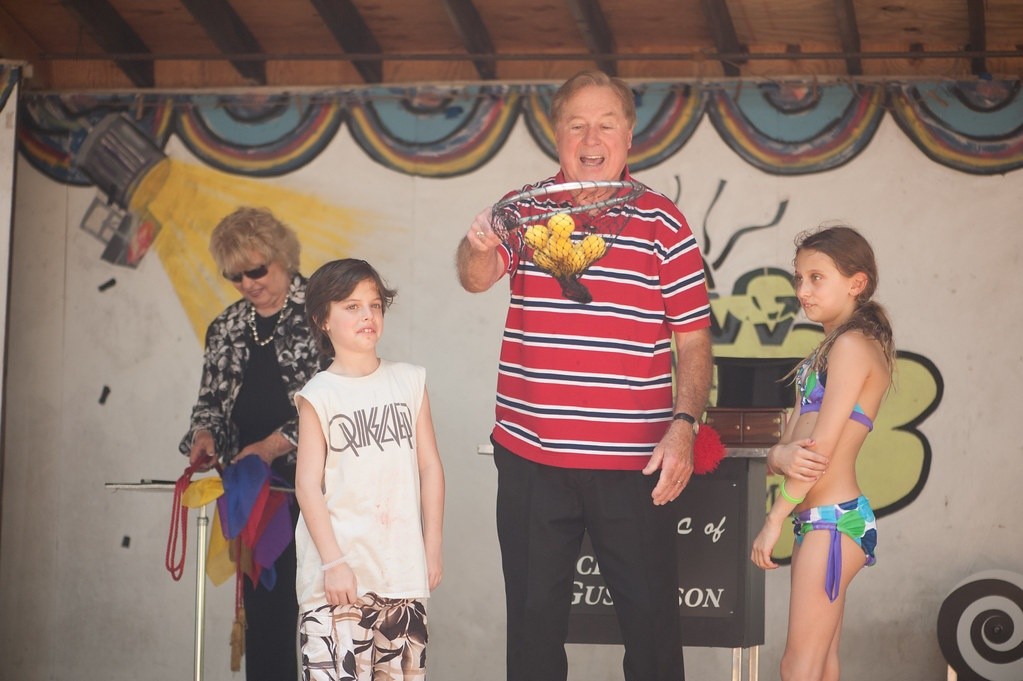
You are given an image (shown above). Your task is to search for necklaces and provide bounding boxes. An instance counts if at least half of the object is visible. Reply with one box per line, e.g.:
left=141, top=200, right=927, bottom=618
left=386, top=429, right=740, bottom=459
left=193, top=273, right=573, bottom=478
left=246, top=300, right=294, bottom=347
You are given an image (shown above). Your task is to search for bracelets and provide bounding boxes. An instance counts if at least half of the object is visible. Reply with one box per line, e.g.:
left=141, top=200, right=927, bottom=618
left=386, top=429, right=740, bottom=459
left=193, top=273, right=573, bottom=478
left=781, top=479, right=805, bottom=505
left=673, top=413, right=695, bottom=424
left=320, top=556, right=347, bottom=571
left=767, top=444, right=781, bottom=477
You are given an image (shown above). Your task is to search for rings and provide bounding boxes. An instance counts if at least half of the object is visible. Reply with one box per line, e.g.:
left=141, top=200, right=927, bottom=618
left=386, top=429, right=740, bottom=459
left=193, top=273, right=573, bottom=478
left=678, top=480, right=683, bottom=484
left=477, top=232, right=484, bottom=237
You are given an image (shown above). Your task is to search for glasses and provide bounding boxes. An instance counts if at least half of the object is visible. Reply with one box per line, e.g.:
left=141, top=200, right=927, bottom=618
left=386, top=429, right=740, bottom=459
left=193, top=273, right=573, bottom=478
left=222, top=254, right=278, bottom=282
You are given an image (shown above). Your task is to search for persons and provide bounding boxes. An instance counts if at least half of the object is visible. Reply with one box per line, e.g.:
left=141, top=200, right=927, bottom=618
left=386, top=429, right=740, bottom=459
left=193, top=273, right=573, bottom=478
left=750, top=225, right=892, bottom=681
left=179, top=208, right=445, bottom=681
left=457, top=73, right=712, bottom=680
